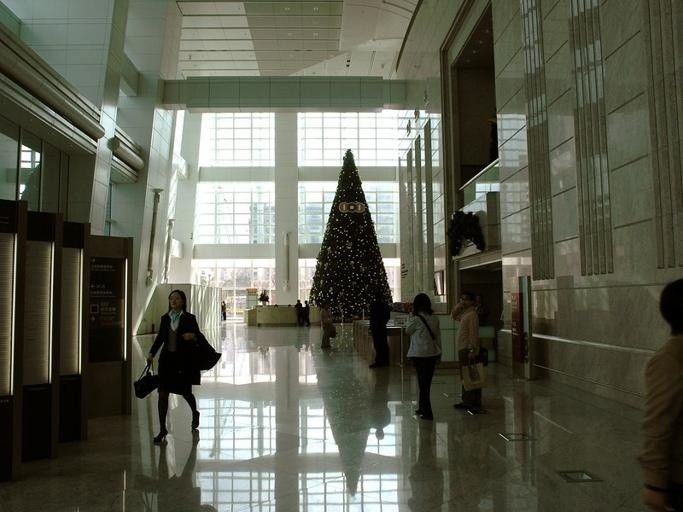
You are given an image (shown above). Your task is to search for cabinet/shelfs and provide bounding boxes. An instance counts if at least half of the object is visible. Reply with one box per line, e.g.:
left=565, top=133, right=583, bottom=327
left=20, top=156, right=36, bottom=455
left=154, top=283, right=222, bottom=333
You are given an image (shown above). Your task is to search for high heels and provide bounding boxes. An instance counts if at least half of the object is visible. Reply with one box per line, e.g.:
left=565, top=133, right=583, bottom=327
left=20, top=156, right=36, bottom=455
left=192, top=412, right=198, bottom=429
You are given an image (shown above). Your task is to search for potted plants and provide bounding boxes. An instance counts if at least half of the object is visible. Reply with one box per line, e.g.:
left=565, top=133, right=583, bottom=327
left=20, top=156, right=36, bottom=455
left=259, top=290, right=269, bottom=305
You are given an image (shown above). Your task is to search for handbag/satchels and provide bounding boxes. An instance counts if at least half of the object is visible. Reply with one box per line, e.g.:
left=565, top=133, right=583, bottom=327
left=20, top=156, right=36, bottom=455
left=458, top=347, right=488, bottom=365
left=133, top=375, right=159, bottom=398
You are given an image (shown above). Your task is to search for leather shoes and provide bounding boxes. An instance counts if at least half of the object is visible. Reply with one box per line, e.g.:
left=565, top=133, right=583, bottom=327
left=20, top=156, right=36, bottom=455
left=154, top=430, right=167, bottom=440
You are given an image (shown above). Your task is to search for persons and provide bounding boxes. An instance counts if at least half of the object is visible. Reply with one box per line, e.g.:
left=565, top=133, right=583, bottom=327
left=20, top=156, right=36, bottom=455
left=642, top=278, right=683, bottom=512
left=451, top=292, right=488, bottom=416
left=304, top=301, right=311, bottom=326
left=295, top=300, right=304, bottom=326
left=320, top=302, right=333, bottom=349
left=369, top=297, right=391, bottom=368
left=222, top=301, right=226, bottom=320
left=147, top=289, right=200, bottom=442
left=405, top=293, right=443, bottom=420
